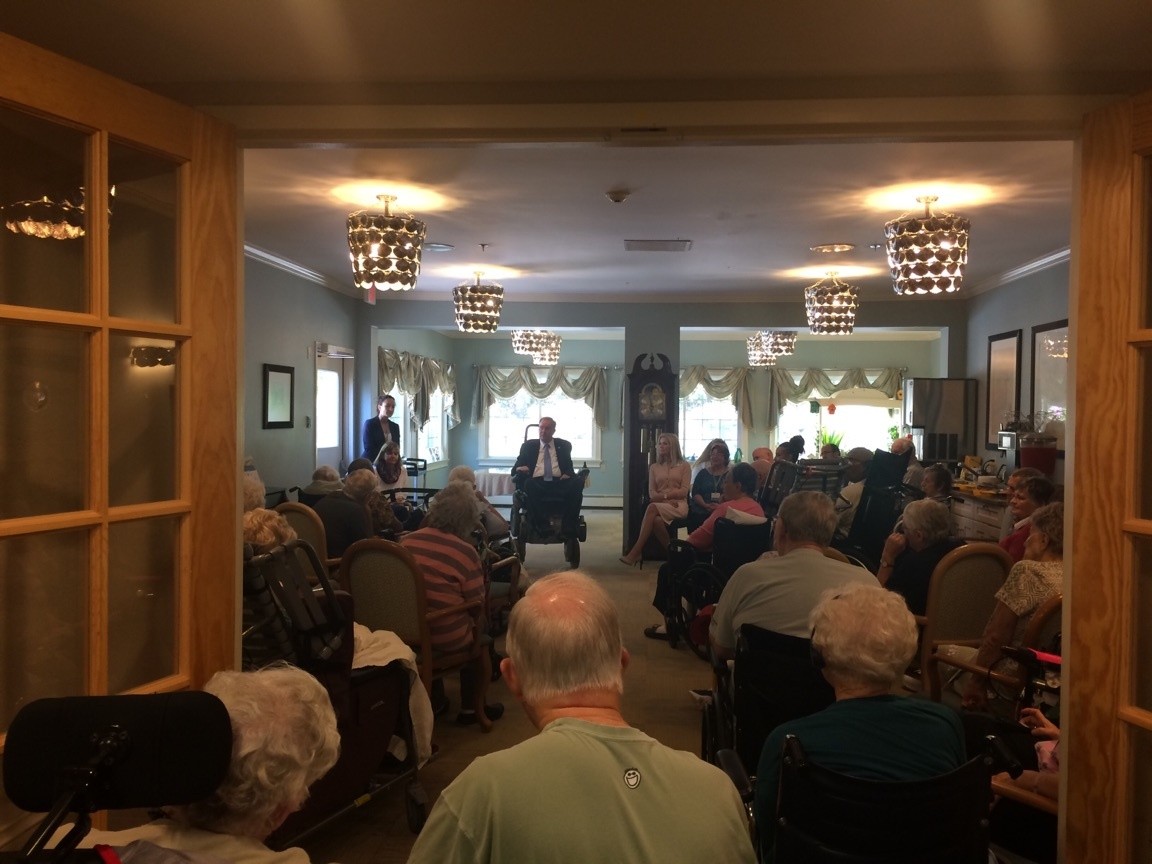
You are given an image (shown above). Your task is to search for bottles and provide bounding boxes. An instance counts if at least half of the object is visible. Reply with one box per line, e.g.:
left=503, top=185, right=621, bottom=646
left=734, top=447, right=742, bottom=464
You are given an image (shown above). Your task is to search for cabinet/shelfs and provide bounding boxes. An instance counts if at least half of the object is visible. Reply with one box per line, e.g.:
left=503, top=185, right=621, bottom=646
left=950, top=495, right=1006, bottom=543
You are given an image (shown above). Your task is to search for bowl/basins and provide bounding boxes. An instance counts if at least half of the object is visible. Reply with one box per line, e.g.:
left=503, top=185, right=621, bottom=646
left=978, top=476, right=996, bottom=484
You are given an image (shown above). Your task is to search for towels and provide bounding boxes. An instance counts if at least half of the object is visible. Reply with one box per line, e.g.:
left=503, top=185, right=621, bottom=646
left=351, top=621, right=434, bottom=771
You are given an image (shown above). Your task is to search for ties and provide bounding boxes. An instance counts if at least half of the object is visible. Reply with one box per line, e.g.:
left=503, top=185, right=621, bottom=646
left=544, top=444, right=553, bottom=481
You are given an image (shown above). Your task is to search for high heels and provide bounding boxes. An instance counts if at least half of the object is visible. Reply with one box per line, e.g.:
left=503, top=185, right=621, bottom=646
left=619, top=556, right=643, bottom=570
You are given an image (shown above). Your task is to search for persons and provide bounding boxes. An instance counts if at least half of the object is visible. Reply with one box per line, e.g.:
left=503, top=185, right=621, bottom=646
left=511, top=417, right=579, bottom=535
left=619, top=433, right=692, bottom=570
left=756, top=584, right=968, bottom=864
left=361, top=395, right=400, bottom=463
left=44, top=660, right=342, bottom=864
left=242, top=441, right=530, bottom=768
left=409, top=571, right=757, bottom=864
left=644, top=434, right=1064, bottom=799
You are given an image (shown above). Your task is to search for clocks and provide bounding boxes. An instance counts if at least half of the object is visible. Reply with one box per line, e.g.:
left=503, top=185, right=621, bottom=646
left=625, top=351, right=679, bottom=558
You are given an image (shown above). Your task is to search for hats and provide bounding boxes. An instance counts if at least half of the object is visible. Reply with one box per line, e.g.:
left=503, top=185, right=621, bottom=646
left=689, top=603, right=718, bottom=645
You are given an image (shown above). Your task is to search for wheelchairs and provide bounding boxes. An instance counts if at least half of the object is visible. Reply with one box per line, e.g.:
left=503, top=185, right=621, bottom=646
left=693, top=622, right=836, bottom=770
left=507, top=424, right=590, bottom=571
left=659, top=516, right=774, bottom=661
left=712, top=733, right=1024, bottom=864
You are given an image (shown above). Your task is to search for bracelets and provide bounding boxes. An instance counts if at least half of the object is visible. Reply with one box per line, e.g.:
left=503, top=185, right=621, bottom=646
left=880, top=559, right=896, bottom=569
left=663, top=492, right=668, bottom=500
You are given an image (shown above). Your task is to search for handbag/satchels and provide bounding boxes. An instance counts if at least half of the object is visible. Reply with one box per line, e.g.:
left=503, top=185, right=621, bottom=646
left=490, top=547, right=512, bottom=581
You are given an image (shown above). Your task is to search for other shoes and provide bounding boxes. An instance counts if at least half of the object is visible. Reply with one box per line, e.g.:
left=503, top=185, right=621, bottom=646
left=690, top=689, right=713, bottom=704
left=536, top=520, right=554, bottom=535
left=645, top=624, right=668, bottom=639
left=564, top=538, right=579, bottom=562
left=457, top=702, right=504, bottom=725
left=434, top=698, right=449, bottom=718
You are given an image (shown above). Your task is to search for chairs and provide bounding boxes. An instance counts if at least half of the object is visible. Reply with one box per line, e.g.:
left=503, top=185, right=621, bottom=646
left=762, top=458, right=1058, bottom=812
left=252, top=502, right=518, bottom=730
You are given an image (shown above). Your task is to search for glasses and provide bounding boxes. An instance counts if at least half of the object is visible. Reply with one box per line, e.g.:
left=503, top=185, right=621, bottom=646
left=821, top=451, right=834, bottom=456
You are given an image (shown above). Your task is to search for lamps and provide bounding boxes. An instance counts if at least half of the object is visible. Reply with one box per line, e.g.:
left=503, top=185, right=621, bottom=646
left=747, top=331, right=796, bottom=366
left=344, top=194, right=427, bottom=288
left=129, top=339, right=175, bottom=369
left=805, top=273, right=858, bottom=333
left=884, top=197, right=971, bottom=296
left=1043, top=336, right=1067, bottom=357
left=512, top=329, right=562, bottom=363
left=452, top=270, right=505, bottom=331
left=2, top=165, right=115, bottom=238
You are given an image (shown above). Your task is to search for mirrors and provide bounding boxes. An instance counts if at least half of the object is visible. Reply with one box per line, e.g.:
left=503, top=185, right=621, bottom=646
left=985, top=329, right=1022, bottom=452
left=262, top=364, right=294, bottom=428
left=1030, top=318, right=1068, bottom=458
